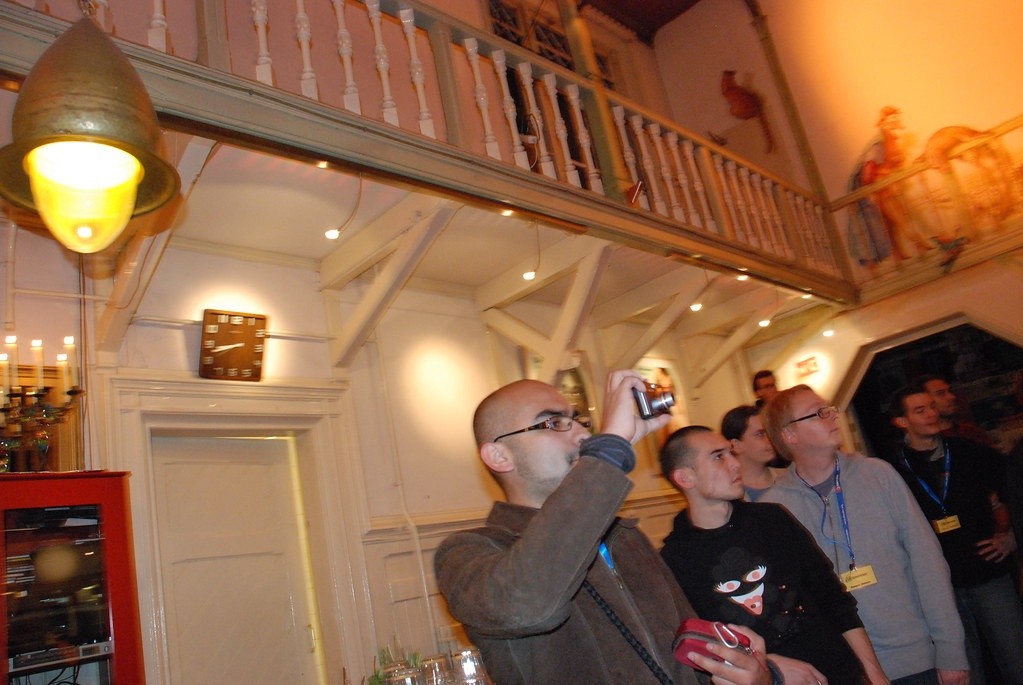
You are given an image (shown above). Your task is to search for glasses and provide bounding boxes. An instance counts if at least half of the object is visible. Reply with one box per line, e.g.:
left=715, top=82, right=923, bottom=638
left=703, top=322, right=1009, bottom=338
left=493, top=411, right=591, bottom=442
left=789, top=405, right=840, bottom=424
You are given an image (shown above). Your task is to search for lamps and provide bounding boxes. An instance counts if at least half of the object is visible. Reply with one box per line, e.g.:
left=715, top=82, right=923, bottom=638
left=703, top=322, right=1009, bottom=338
left=0, top=0, right=182, bottom=254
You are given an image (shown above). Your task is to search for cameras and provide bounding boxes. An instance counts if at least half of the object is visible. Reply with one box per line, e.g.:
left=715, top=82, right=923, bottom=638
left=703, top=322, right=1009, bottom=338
left=632, top=386, right=676, bottom=419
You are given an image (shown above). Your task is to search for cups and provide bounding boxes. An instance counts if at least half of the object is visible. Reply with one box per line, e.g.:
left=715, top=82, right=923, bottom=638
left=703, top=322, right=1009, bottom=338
left=384, top=660, right=409, bottom=673
left=422, top=653, right=455, bottom=685
left=452, top=646, right=494, bottom=685
left=385, top=667, right=422, bottom=685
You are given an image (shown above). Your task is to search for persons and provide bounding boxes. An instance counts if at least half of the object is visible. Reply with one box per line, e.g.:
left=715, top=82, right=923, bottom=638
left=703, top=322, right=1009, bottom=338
left=648, top=375, right=1023, bottom=685
left=753, top=371, right=780, bottom=408
left=719, top=405, right=787, bottom=502
left=435, top=370, right=784, bottom=685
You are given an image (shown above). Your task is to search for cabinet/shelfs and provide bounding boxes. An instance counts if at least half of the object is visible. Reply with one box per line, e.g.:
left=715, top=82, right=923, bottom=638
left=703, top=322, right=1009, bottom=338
left=0, top=468, right=147, bottom=685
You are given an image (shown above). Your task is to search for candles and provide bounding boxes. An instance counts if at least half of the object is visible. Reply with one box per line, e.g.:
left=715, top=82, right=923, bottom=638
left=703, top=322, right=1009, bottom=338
left=6, top=335, right=20, bottom=387
left=31, top=340, right=45, bottom=390
left=0, top=353, right=10, bottom=404
left=55, top=354, right=70, bottom=402
left=63, top=335, right=80, bottom=387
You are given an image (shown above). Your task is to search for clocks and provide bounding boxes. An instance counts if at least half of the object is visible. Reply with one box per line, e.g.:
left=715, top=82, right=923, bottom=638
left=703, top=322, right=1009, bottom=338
left=198, top=308, right=270, bottom=383
